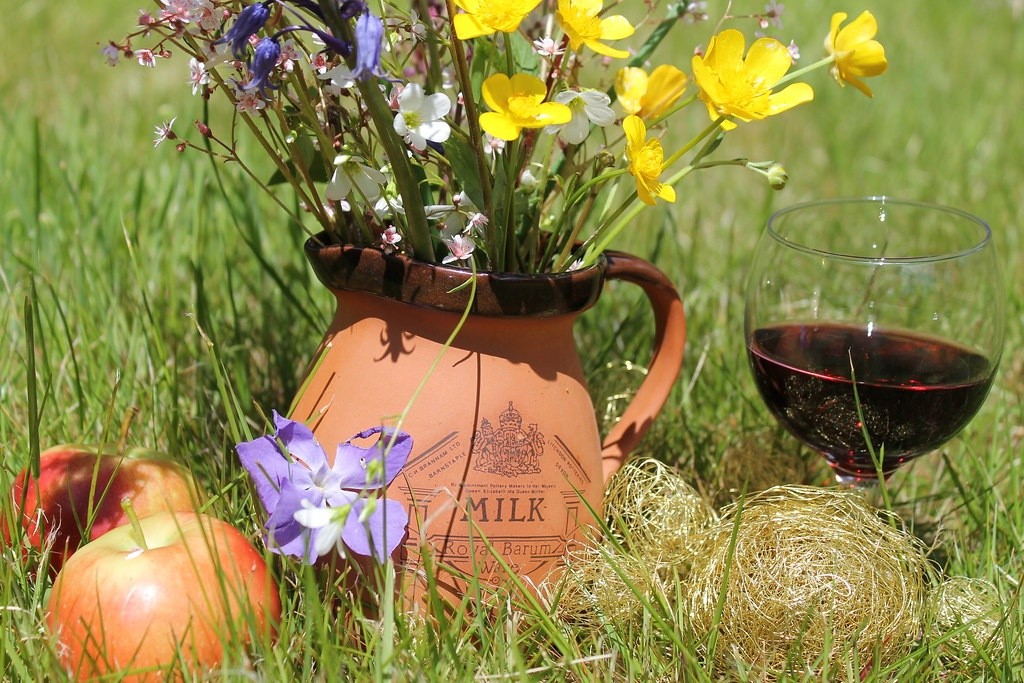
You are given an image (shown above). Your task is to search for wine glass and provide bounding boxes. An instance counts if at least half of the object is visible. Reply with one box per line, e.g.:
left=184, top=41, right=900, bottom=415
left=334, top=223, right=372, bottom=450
left=745, top=195, right=1005, bottom=531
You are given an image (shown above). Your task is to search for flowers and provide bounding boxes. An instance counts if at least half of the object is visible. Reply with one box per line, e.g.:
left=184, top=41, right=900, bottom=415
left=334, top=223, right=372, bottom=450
left=101, top=0, right=888, bottom=567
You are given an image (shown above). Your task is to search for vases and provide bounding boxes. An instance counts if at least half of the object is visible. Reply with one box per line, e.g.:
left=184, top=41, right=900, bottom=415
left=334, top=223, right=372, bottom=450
left=284, top=225, right=688, bottom=650
